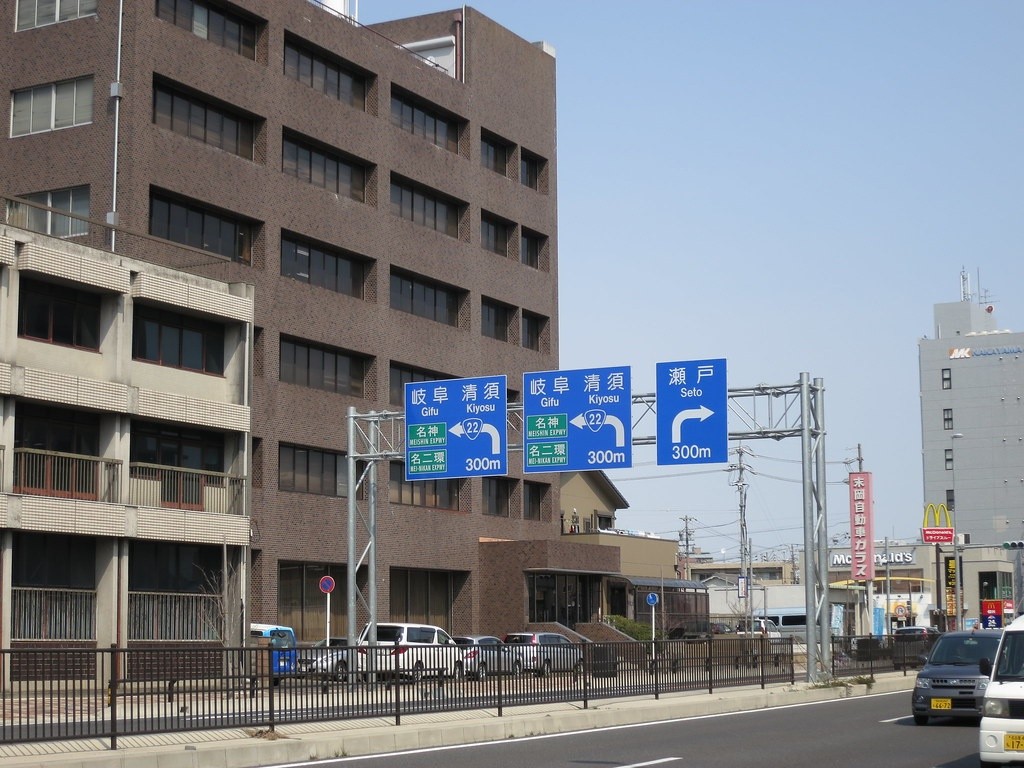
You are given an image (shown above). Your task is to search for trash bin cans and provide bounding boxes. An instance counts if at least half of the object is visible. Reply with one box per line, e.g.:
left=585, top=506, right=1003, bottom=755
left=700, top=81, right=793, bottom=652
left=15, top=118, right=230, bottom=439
left=857, top=638, right=879, bottom=661
left=592, top=646, right=617, bottom=677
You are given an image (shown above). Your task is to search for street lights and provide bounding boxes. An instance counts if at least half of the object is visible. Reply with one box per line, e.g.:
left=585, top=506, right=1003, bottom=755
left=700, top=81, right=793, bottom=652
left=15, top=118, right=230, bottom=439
left=951, top=433, right=964, bottom=631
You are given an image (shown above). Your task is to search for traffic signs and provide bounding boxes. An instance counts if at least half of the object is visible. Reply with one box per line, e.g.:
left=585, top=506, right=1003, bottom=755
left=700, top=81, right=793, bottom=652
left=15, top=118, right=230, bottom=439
left=403, top=374, right=509, bottom=482
left=655, top=357, right=728, bottom=466
left=522, top=365, right=632, bottom=473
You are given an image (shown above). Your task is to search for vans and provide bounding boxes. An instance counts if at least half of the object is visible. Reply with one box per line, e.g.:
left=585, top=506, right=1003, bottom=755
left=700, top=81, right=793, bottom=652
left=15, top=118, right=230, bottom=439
left=912, top=629, right=1009, bottom=726
left=752, top=607, right=821, bottom=644
left=978, top=614, right=1024, bottom=768
left=250, top=623, right=297, bottom=686
left=893, top=626, right=942, bottom=670
left=737, top=618, right=781, bottom=644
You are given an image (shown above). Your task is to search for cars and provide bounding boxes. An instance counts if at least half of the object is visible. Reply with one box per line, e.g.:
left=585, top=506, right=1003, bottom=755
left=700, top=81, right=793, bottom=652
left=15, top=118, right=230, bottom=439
left=711, top=622, right=731, bottom=635
left=356, top=622, right=467, bottom=683
left=501, top=632, right=585, bottom=677
left=296, top=637, right=357, bottom=683
left=451, top=635, right=524, bottom=682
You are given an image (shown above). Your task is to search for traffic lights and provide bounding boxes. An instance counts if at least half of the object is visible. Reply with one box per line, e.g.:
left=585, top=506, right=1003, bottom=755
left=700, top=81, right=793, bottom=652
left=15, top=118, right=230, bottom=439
left=1004, top=541, right=1024, bottom=549
